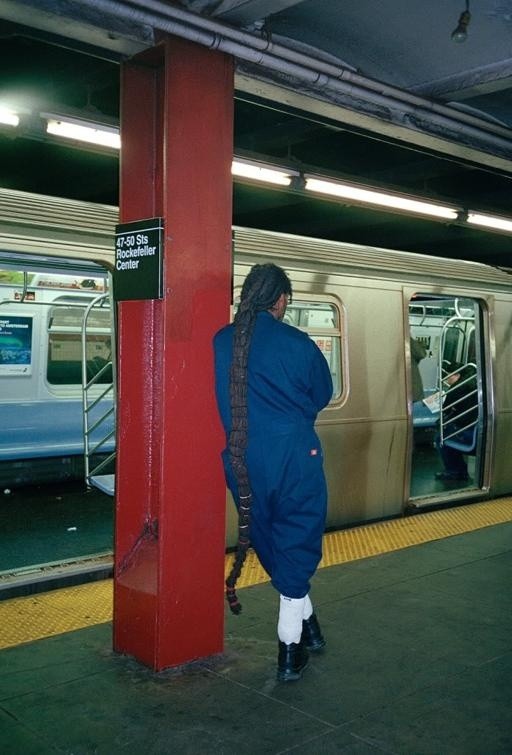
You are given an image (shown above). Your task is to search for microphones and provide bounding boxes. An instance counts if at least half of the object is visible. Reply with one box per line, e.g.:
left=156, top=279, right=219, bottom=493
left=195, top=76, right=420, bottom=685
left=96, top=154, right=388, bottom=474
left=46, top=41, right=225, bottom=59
left=89, top=472, right=115, bottom=496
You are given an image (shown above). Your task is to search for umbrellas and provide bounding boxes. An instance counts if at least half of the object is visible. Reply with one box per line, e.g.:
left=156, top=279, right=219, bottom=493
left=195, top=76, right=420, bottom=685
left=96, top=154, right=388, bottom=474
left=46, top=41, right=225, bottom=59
left=437, top=467, right=468, bottom=484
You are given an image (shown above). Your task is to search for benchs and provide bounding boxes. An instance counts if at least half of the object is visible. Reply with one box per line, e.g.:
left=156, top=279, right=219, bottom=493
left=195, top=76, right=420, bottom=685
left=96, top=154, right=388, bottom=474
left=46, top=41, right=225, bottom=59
left=412, top=386, right=443, bottom=429
left=89, top=472, right=115, bottom=496
left=440, top=420, right=480, bottom=456
left=0, top=397, right=116, bottom=459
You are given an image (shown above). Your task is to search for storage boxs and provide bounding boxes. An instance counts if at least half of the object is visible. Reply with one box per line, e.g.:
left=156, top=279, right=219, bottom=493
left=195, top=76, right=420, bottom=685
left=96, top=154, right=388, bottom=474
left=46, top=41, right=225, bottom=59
left=0, top=397, right=116, bottom=459
left=412, top=386, right=443, bottom=429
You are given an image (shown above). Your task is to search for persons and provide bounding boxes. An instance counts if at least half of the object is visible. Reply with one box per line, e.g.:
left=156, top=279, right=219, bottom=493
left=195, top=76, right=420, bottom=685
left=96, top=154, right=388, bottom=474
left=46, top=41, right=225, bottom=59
left=208, top=258, right=339, bottom=686
left=434, top=360, right=478, bottom=479
left=411, top=338, right=428, bottom=404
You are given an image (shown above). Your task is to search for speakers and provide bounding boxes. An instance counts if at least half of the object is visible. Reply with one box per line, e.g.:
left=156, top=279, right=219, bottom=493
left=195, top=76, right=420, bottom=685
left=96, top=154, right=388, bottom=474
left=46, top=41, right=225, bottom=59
left=277, top=640, right=310, bottom=679
left=302, top=610, right=327, bottom=649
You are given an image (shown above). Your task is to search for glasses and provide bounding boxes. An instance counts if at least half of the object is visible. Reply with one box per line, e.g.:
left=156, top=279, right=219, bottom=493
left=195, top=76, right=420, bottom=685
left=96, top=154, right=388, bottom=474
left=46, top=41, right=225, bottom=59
left=448, top=1, right=475, bottom=44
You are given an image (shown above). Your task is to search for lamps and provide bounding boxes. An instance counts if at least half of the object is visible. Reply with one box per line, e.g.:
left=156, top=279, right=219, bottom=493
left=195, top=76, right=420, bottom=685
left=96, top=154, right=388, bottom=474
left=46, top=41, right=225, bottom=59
left=300, top=177, right=464, bottom=221
left=231, top=161, right=298, bottom=189
left=464, top=211, right=511, bottom=232
left=440, top=420, right=480, bottom=456
left=32, top=116, right=121, bottom=150
left=0, top=111, right=32, bottom=129
left=448, top=1, right=475, bottom=44
